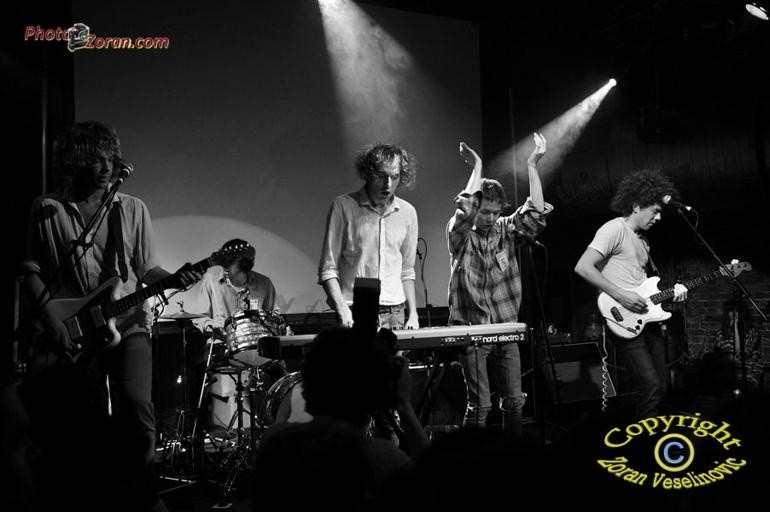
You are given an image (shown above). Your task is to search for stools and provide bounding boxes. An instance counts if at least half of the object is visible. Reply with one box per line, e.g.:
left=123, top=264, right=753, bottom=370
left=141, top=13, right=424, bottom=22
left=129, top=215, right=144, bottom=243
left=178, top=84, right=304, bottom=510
left=201, top=360, right=254, bottom=446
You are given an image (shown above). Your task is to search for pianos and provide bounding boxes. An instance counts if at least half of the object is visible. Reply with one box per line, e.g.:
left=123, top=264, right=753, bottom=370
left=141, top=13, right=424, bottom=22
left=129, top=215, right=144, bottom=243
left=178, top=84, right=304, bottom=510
left=258, top=322, right=527, bottom=360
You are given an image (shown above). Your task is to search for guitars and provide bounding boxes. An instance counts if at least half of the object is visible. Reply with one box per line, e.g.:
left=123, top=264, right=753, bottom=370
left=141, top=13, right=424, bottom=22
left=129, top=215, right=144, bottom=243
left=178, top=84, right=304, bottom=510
left=597, top=262, right=753, bottom=339
left=29, top=244, right=250, bottom=369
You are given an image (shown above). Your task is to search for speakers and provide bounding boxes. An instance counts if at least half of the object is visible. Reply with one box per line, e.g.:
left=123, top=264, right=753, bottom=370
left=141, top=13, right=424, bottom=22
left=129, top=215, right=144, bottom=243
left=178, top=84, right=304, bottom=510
left=542, top=342, right=618, bottom=404
left=409, top=363, right=468, bottom=425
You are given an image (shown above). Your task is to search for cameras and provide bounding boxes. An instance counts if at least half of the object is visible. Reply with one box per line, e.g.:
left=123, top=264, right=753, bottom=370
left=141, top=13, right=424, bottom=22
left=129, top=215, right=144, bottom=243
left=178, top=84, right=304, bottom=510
left=353, top=278, right=397, bottom=378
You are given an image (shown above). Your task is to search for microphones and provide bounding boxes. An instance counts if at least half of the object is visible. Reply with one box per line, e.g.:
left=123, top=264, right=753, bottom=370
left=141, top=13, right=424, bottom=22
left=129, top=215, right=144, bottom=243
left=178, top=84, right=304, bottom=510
left=663, top=195, right=692, bottom=212
left=507, top=224, right=540, bottom=245
left=111, top=162, right=135, bottom=193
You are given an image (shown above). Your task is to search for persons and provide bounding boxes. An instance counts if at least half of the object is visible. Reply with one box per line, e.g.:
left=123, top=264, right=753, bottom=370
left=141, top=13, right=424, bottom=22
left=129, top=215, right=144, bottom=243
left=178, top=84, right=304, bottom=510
left=316, top=142, right=418, bottom=446
left=189, top=240, right=287, bottom=432
left=444, top=131, right=556, bottom=438
left=571, top=170, right=688, bottom=424
left=18, top=122, right=204, bottom=465
left=3, top=298, right=769, bottom=510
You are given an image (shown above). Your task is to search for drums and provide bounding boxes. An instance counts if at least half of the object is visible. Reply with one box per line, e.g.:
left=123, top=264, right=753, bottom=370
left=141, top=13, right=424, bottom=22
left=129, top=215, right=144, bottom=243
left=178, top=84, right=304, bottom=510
left=224, top=311, right=286, bottom=366
left=260, top=372, right=313, bottom=437
left=207, top=365, right=286, bottom=429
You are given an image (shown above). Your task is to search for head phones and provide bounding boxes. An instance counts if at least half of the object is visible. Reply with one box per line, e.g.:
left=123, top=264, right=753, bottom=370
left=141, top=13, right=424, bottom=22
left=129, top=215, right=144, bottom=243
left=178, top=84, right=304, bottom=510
left=239, top=258, right=254, bottom=272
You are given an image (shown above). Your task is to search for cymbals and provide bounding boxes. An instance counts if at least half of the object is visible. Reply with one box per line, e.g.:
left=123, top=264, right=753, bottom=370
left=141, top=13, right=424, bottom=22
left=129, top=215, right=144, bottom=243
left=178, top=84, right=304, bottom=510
left=159, top=314, right=207, bottom=318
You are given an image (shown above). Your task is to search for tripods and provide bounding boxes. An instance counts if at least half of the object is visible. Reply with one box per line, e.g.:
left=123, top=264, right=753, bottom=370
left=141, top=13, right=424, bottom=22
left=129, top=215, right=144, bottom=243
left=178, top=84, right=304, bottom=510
left=164, top=327, right=219, bottom=452
left=222, top=376, right=264, bottom=449
